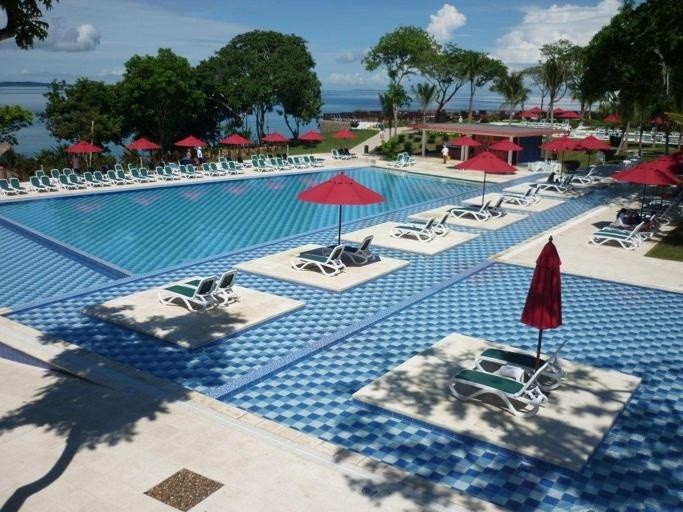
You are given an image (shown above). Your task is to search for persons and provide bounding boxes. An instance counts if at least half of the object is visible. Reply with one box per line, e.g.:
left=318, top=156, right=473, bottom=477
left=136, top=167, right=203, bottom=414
left=196, top=147, right=203, bottom=164
left=610, top=209, right=656, bottom=232
left=441, top=144, right=449, bottom=164
left=337, top=145, right=351, bottom=155
left=72, top=153, right=80, bottom=174
left=186, top=148, right=192, bottom=163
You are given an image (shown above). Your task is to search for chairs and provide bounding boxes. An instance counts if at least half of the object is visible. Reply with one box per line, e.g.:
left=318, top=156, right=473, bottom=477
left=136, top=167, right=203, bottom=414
left=450, top=335, right=568, bottom=416
left=157, top=269, right=240, bottom=314
left=389, top=153, right=682, bottom=273
left=292, top=235, right=377, bottom=276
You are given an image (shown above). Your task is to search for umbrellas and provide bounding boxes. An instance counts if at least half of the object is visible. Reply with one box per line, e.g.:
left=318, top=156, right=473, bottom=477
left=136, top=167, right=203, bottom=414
left=520, top=236, right=562, bottom=385
left=220, top=133, right=250, bottom=164
left=127, top=137, right=162, bottom=167
left=518, top=106, right=683, bottom=216
left=454, top=151, right=519, bottom=207
left=488, top=139, right=524, bottom=162
left=63, top=140, right=104, bottom=153
left=297, top=131, right=326, bottom=155
left=448, top=135, right=482, bottom=159
left=173, top=135, right=209, bottom=149
left=261, top=132, right=291, bottom=154
left=297, top=172, right=384, bottom=246
left=331, top=128, right=356, bottom=155
